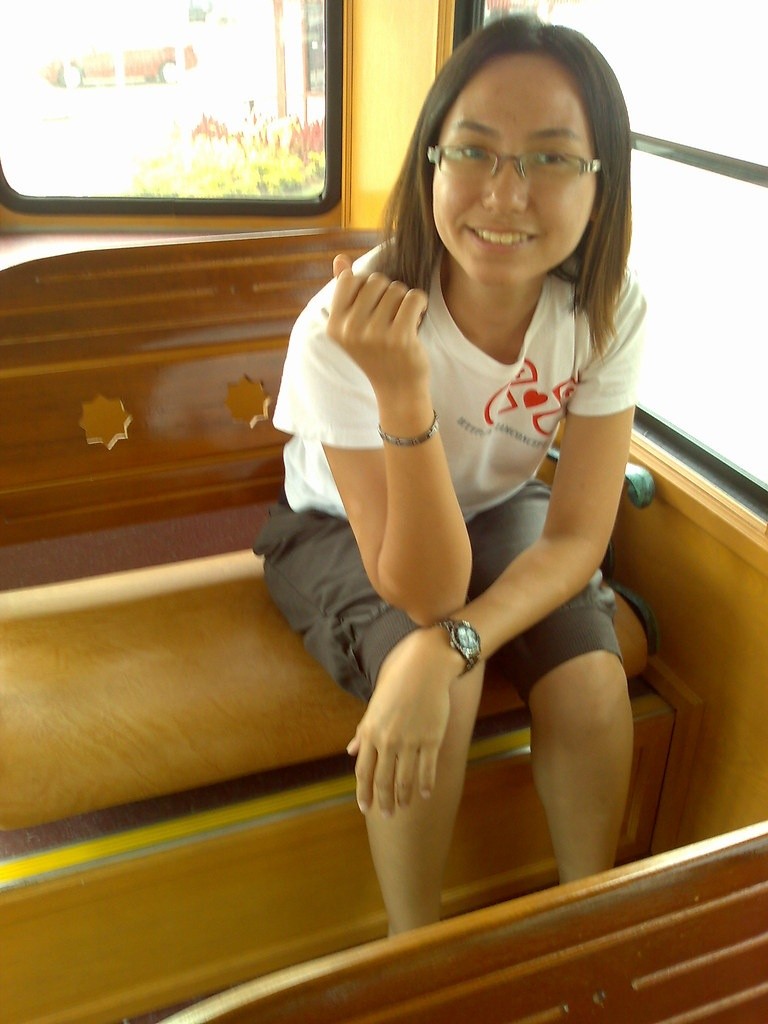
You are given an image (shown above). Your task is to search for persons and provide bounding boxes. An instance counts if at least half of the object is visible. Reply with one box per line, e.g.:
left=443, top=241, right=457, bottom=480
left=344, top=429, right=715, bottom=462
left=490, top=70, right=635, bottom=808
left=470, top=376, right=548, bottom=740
left=252, top=12, right=650, bottom=939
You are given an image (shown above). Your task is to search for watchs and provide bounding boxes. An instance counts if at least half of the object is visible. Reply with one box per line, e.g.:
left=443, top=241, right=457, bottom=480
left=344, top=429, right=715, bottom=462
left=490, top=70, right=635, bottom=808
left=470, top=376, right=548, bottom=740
left=435, top=618, right=481, bottom=676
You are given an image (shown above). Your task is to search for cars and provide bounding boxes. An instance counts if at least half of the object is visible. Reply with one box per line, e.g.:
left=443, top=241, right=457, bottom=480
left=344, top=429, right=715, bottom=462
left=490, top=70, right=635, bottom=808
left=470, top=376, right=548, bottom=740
left=39, top=42, right=199, bottom=88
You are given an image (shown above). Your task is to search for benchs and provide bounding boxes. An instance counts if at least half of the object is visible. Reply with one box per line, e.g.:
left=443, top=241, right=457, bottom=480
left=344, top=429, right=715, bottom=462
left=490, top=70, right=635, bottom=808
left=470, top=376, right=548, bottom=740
left=0, top=220, right=768, bottom=1024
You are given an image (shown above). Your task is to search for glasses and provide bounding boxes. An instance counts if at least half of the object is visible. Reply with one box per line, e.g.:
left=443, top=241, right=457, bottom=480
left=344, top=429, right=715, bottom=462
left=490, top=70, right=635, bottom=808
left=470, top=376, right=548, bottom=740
left=426, top=144, right=601, bottom=189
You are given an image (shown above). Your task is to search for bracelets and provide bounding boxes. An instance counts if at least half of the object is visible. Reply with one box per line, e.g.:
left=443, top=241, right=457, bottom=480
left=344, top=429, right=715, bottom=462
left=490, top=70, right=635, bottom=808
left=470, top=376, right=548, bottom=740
left=378, top=410, right=440, bottom=447
